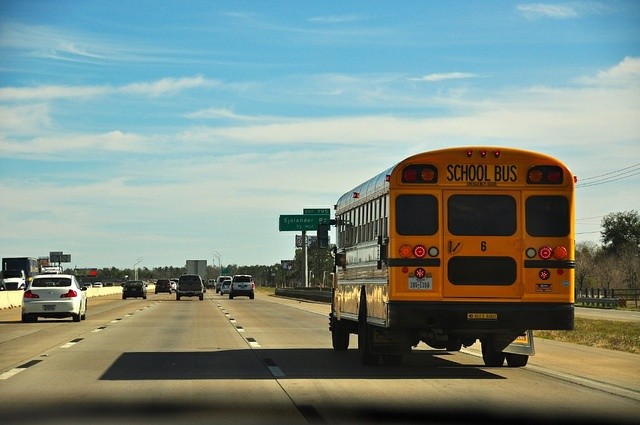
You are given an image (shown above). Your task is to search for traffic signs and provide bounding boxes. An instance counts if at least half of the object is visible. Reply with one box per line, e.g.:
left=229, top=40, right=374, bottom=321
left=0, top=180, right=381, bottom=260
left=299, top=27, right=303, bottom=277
left=280, top=209, right=331, bottom=231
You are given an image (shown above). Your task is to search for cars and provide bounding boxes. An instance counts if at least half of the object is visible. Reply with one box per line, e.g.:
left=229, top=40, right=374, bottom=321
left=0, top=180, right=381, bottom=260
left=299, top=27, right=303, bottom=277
left=221, top=280, right=232, bottom=296
left=23, top=275, right=88, bottom=323
left=123, top=281, right=148, bottom=300
left=171, top=281, right=177, bottom=292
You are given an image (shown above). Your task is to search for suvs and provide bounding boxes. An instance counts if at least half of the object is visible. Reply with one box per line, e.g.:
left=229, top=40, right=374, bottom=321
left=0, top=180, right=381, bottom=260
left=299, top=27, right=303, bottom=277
left=217, top=276, right=233, bottom=294
left=94, top=282, right=104, bottom=288
left=177, top=275, right=205, bottom=301
left=230, top=275, right=256, bottom=300
left=81, top=283, right=93, bottom=291
left=156, top=280, right=173, bottom=294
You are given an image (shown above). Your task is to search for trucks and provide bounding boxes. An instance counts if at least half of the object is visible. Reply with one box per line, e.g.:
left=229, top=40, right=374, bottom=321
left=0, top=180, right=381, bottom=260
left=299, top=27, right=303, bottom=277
left=0, top=258, right=39, bottom=291
left=41, top=266, right=64, bottom=275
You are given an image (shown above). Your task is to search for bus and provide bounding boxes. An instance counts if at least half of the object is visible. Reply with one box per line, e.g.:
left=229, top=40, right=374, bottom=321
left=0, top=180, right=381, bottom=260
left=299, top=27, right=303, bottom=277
left=317, top=145, right=576, bottom=368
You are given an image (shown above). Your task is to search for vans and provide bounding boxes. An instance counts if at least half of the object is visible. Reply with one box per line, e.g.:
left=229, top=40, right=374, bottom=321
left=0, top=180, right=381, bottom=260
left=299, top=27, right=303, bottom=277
left=208, top=279, right=217, bottom=290
